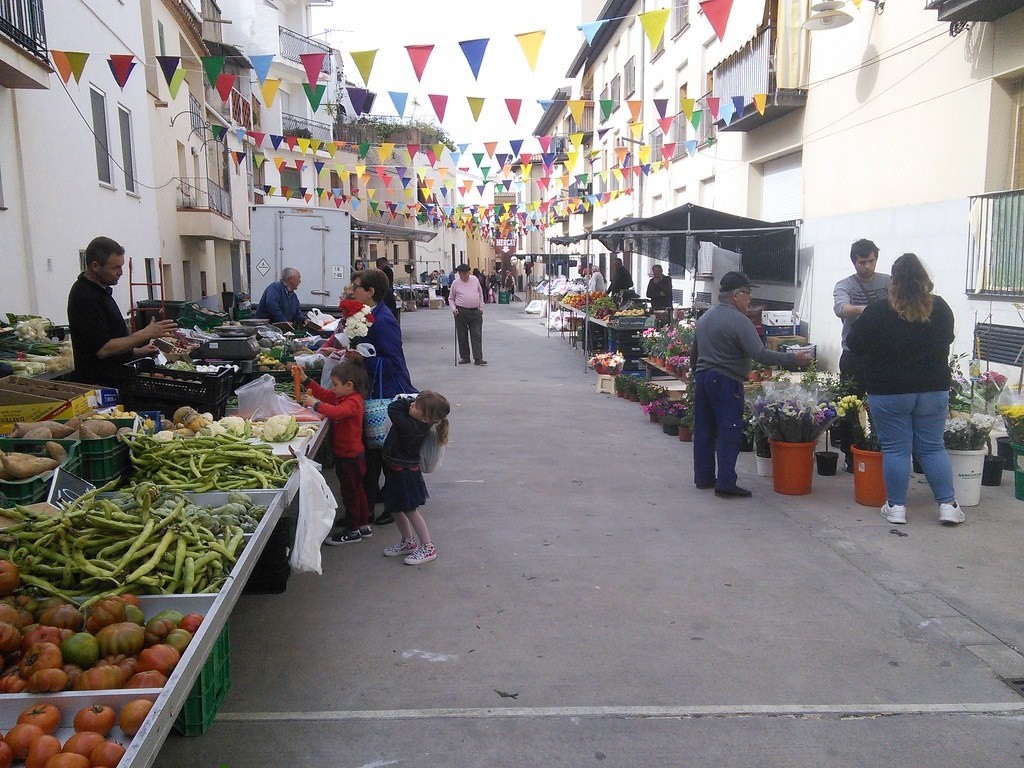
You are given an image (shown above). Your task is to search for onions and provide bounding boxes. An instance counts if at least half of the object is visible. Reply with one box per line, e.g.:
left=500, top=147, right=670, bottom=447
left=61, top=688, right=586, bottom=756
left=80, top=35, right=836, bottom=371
left=14, top=319, right=46, bottom=339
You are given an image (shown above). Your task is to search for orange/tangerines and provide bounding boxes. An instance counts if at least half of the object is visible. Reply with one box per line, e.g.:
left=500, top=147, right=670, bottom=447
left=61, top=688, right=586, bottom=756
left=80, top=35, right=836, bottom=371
left=569, top=291, right=607, bottom=304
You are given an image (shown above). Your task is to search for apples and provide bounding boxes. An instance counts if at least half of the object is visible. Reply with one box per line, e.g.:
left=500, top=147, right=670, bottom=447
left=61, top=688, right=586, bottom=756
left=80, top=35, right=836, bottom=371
left=575, top=294, right=594, bottom=309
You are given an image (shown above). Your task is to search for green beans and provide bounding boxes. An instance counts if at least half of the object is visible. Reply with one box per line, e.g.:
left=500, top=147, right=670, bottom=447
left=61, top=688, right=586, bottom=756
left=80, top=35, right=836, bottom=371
left=0, top=476, right=284, bottom=604
left=118, top=425, right=299, bottom=493
left=588, top=297, right=618, bottom=314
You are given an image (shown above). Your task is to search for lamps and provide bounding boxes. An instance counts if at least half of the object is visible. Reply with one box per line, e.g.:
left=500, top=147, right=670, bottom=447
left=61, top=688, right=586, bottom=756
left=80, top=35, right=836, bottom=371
left=170, top=110, right=231, bottom=155
left=802, top=0, right=885, bottom=31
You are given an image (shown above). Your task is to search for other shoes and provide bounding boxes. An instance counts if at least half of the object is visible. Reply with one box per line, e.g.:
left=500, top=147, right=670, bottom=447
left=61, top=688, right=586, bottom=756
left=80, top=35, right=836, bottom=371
left=492, top=301, right=496, bottom=303
left=696, top=474, right=718, bottom=488
left=714, top=485, right=751, bottom=498
left=376, top=511, right=394, bottom=524
left=847, top=465, right=854, bottom=473
left=460, top=357, right=470, bottom=364
left=335, top=511, right=374, bottom=527
left=475, top=359, right=487, bottom=364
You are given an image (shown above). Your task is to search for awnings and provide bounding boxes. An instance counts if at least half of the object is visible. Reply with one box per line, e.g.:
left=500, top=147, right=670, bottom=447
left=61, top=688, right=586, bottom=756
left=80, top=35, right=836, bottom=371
left=351, top=215, right=438, bottom=242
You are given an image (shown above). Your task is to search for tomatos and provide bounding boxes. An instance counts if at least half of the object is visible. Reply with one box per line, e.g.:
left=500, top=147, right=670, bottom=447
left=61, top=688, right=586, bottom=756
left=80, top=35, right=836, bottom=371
left=0, top=561, right=204, bottom=768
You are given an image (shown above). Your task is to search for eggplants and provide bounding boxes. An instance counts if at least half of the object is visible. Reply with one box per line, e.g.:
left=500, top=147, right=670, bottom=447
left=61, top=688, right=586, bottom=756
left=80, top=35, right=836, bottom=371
left=594, top=308, right=622, bottom=319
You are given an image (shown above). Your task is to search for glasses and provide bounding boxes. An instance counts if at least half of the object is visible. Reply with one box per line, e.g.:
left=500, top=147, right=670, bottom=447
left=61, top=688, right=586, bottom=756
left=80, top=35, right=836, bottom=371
left=734, top=289, right=752, bottom=297
left=351, top=283, right=376, bottom=292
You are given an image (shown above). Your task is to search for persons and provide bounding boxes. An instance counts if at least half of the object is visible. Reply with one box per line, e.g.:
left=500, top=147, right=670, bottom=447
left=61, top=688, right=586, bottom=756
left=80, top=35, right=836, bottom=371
left=481, top=270, right=489, bottom=304
left=381, top=390, right=450, bottom=566
left=253, top=267, right=306, bottom=324
left=689, top=271, right=814, bottom=497
left=355, top=260, right=363, bottom=270
left=440, top=269, right=449, bottom=305
left=473, top=268, right=484, bottom=291
left=449, top=264, right=487, bottom=365
left=490, top=270, right=497, bottom=303
left=344, top=268, right=419, bottom=526
left=846, top=253, right=965, bottom=524
left=315, top=283, right=354, bottom=356
left=67, top=236, right=178, bottom=412
left=430, top=270, right=439, bottom=281
left=376, top=257, right=393, bottom=291
left=646, top=265, right=672, bottom=308
left=504, top=270, right=516, bottom=301
left=420, top=271, right=429, bottom=282
left=589, top=265, right=605, bottom=292
left=448, top=268, right=457, bottom=287
left=291, top=362, right=372, bottom=545
left=602, top=258, right=633, bottom=296
left=832, top=239, right=892, bottom=472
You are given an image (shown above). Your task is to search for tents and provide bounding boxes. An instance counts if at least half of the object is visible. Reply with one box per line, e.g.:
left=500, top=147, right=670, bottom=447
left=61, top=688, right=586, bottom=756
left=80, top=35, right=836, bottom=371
left=547, top=201, right=799, bottom=373
left=545, top=217, right=647, bottom=336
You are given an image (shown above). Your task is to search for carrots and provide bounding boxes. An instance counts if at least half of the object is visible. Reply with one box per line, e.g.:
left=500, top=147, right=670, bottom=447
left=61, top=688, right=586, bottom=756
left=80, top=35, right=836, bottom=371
left=283, top=365, right=325, bottom=421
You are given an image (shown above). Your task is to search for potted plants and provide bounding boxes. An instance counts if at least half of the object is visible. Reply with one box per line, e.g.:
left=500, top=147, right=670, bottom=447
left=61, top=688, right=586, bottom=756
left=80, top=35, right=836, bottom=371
left=613, top=374, right=650, bottom=406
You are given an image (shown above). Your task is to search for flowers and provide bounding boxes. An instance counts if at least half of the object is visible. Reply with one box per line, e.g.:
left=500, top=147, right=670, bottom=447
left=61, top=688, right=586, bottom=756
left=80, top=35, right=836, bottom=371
left=635, top=317, right=1024, bottom=457
left=587, top=351, right=627, bottom=376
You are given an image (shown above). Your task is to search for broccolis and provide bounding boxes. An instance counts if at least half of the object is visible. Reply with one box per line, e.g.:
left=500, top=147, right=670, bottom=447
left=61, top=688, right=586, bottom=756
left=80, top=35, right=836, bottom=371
left=152, top=414, right=298, bottom=442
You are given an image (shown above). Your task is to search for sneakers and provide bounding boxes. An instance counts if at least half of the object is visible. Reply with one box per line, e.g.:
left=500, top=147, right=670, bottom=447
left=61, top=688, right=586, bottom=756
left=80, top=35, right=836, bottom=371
left=940, top=497, right=966, bottom=524
left=359, top=524, right=372, bottom=538
left=880, top=499, right=907, bottom=524
left=403, top=543, right=436, bottom=565
left=322, top=527, right=361, bottom=545
left=383, top=537, right=418, bottom=557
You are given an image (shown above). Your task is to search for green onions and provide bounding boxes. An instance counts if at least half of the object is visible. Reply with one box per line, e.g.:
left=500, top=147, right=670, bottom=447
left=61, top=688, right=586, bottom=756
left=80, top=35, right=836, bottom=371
left=0, top=337, right=75, bottom=375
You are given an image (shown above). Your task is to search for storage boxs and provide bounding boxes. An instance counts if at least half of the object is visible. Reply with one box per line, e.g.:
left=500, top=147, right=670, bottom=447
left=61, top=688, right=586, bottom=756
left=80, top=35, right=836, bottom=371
left=0, top=300, right=232, bottom=507
left=565, top=317, right=583, bottom=330
left=173, top=617, right=233, bottom=738
left=242, top=518, right=290, bottom=593
left=753, top=310, right=817, bottom=371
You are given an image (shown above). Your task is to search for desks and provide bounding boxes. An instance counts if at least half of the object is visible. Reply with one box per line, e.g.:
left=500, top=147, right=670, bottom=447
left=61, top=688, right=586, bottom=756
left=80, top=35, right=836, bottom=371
left=558, top=301, right=608, bottom=357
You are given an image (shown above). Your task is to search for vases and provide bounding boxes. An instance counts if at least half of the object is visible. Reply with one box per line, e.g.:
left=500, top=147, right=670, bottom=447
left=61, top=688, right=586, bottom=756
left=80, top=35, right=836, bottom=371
left=648, top=355, right=1014, bottom=487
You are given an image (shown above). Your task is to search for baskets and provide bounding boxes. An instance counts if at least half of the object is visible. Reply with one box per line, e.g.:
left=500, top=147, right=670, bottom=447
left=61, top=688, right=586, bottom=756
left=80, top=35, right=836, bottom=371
left=121, top=358, right=231, bottom=436
left=183, top=301, right=229, bottom=328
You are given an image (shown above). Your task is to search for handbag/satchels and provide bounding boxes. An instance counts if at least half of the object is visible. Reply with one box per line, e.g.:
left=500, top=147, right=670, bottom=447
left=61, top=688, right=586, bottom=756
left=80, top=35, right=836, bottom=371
left=319, top=344, right=377, bottom=390
left=387, top=393, right=447, bottom=473
left=308, top=307, right=336, bottom=327
left=363, top=357, right=399, bottom=449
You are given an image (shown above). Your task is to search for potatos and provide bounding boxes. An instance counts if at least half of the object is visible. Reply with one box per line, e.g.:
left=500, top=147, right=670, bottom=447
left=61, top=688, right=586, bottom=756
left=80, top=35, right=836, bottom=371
left=259, top=350, right=324, bottom=372
left=0, top=416, right=134, bottom=478
left=614, top=308, right=644, bottom=316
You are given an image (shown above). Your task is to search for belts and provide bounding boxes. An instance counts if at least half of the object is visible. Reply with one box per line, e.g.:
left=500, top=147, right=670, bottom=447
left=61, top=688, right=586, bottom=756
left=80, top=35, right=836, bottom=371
left=458, top=306, right=478, bottom=310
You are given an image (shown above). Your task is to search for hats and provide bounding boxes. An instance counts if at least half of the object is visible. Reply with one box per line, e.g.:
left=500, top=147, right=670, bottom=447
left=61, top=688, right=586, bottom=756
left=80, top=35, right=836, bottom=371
left=719, top=272, right=751, bottom=292
left=457, top=264, right=470, bottom=271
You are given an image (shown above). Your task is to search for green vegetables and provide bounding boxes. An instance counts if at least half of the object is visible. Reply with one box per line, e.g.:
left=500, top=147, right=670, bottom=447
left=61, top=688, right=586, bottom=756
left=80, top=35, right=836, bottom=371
left=172, top=360, right=196, bottom=371
left=5, top=313, right=52, bottom=329
